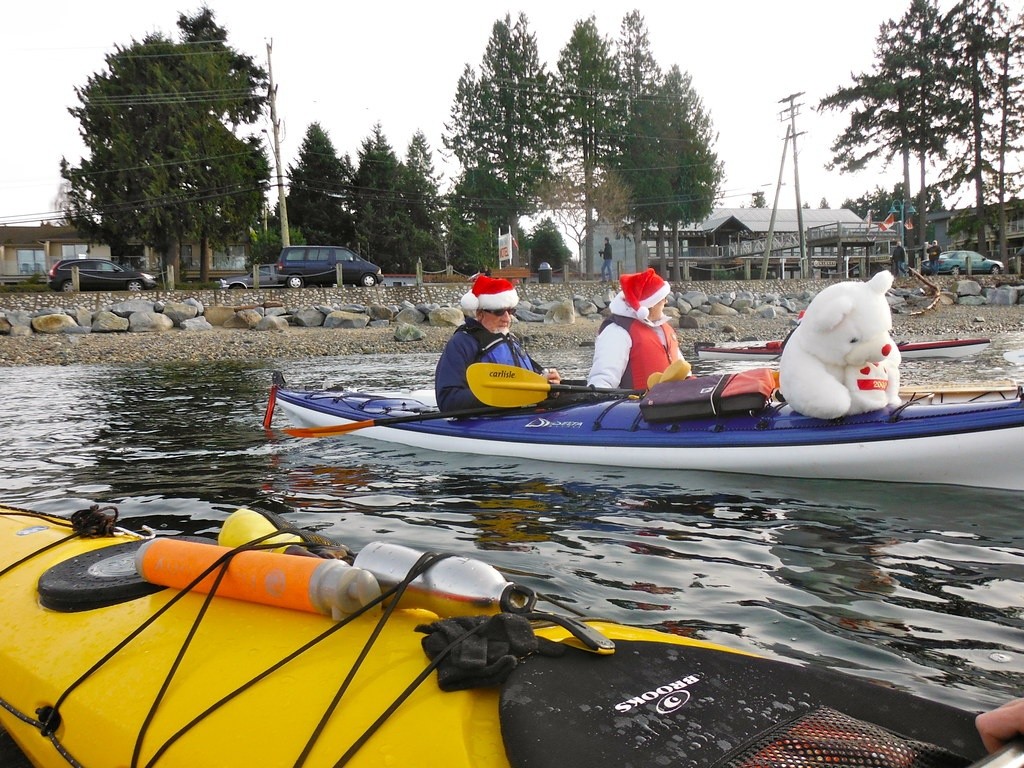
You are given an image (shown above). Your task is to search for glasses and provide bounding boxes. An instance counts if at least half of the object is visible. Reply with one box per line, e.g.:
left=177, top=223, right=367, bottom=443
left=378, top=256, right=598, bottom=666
left=484, top=308, right=517, bottom=315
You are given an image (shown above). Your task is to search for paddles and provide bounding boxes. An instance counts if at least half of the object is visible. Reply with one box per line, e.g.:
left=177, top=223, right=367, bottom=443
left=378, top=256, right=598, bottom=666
left=281, top=396, right=571, bottom=439
left=464, top=361, right=779, bottom=413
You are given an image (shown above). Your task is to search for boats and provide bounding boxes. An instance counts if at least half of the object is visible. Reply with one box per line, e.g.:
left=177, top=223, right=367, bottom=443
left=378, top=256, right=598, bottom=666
left=0, top=504, right=1024, bottom=767
left=693, top=338, right=990, bottom=361
left=264, top=369, right=1024, bottom=490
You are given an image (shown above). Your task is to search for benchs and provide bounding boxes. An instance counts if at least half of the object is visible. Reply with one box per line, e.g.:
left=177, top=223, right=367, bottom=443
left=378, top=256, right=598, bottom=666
left=488, top=268, right=530, bottom=284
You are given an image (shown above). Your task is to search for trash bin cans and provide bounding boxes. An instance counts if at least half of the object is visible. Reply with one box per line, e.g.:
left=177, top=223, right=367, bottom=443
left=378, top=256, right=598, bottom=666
left=539, top=263, right=554, bottom=285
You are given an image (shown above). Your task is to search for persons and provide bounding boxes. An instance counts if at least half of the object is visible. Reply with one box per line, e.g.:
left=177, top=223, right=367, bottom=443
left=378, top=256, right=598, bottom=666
left=435, top=276, right=561, bottom=413
left=598, top=236, right=613, bottom=283
left=926, top=240, right=941, bottom=277
left=890, top=241, right=907, bottom=276
left=974, top=698, right=1024, bottom=754
left=587, top=267, right=693, bottom=389
left=484, top=268, right=491, bottom=276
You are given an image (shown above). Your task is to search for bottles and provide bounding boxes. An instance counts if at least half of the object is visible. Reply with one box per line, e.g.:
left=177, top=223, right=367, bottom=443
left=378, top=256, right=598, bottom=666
left=217, top=509, right=307, bottom=557
left=353, top=542, right=538, bottom=615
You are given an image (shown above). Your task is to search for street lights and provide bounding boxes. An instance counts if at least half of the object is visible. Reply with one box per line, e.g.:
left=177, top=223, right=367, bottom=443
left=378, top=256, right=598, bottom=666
left=262, top=129, right=290, bottom=247
left=887, top=200, right=916, bottom=276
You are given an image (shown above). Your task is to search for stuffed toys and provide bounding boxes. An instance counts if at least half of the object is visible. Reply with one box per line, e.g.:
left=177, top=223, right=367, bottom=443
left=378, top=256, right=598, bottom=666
left=779, top=270, right=902, bottom=419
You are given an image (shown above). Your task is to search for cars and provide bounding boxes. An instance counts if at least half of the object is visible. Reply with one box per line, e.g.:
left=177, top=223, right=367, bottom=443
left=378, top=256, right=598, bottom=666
left=47, top=259, right=159, bottom=292
left=223, top=264, right=285, bottom=289
left=921, top=250, right=1004, bottom=276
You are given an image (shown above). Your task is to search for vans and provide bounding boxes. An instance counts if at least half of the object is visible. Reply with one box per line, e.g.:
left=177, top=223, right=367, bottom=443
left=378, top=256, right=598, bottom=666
left=276, top=246, right=384, bottom=289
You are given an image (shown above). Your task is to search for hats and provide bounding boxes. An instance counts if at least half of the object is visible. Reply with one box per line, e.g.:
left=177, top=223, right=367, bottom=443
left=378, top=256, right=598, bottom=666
left=619, top=268, right=670, bottom=321
left=462, top=275, right=520, bottom=311
